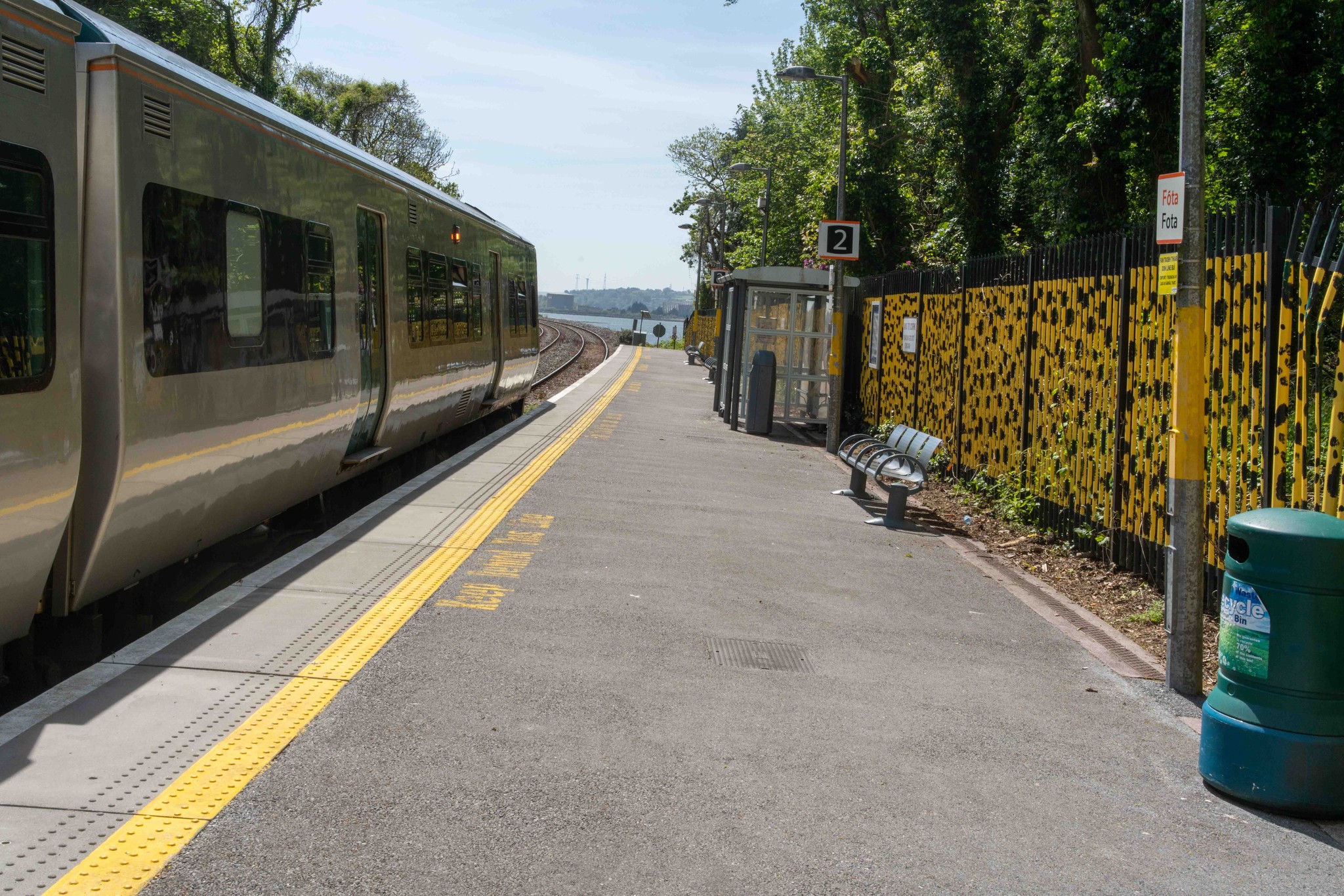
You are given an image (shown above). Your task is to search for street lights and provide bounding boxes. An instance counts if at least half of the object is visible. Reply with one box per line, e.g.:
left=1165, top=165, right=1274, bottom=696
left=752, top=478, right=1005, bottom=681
left=776, top=65, right=850, bottom=454
left=677, top=223, right=705, bottom=346
left=726, top=162, right=773, bottom=314
left=694, top=198, right=726, bottom=358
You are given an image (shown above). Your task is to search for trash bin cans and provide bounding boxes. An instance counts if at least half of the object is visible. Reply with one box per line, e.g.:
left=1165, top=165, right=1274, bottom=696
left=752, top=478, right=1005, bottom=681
left=1194, top=507, right=1344, bottom=821
left=745, top=351, right=777, bottom=436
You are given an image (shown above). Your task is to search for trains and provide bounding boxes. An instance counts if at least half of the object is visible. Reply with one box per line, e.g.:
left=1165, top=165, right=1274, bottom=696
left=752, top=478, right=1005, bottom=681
left=0, top=0, right=541, bottom=653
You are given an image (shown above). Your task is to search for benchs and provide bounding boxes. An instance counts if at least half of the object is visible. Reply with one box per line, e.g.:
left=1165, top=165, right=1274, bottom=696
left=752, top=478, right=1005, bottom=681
left=831, top=424, right=947, bottom=530
